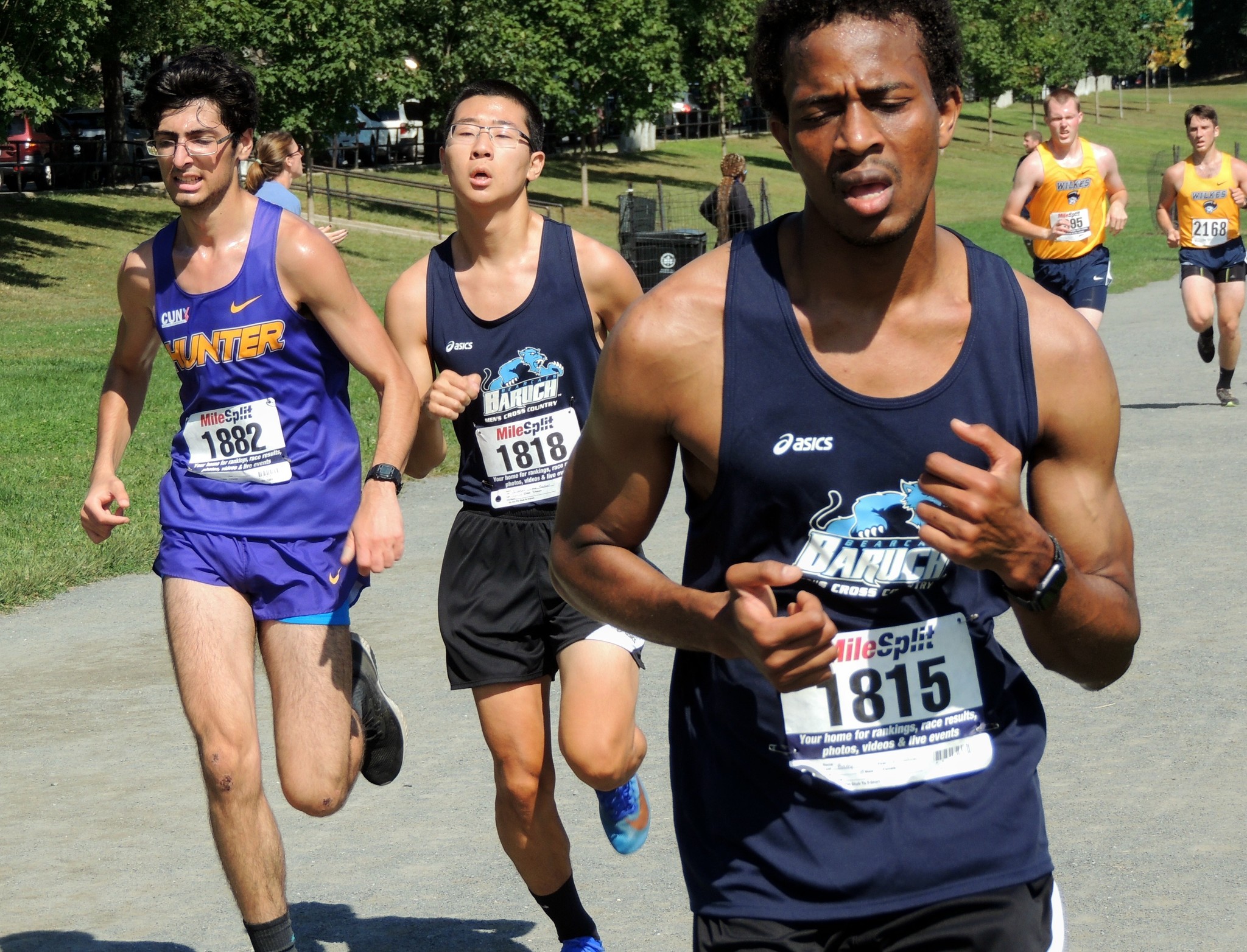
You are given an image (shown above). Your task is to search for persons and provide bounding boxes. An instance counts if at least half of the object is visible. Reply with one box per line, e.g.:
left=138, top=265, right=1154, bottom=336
left=1156, top=104, right=1247, bottom=407
left=548, top=2, right=1145, bottom=951
left=699, top=153, right=755, bottom=250
left=383, top=82, right=674, bottom=951
left=246, top=129, right=350, bottom=247
left=79, top=45, right=420, bottom=951
left=1000, top=89, right=1129, bottom=331
left=237, top=137, right=258, bottom=192
left=1011, top=130, right=1042, bottom=260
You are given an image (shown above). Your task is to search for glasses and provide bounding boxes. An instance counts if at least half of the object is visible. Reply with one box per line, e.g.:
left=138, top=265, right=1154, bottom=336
left=445, top=120, right=537, bottom=152
left=285, top=145, right=305, bottom=159
left=144, top=132, right=235, bottom=157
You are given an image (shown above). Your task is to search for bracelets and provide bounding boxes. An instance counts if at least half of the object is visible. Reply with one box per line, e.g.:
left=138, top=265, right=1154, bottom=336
left=1240, top=198, right=1247, bottom=209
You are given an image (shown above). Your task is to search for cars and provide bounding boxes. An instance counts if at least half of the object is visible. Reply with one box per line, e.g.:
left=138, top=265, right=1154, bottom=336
left=1114, top=64, right=1180, bottom=88
left=548, top=88, right=767, bottom=147
left=315, top=97, right=427, bottom=165
left=0, top=108, right=57, bottom=191
left=49, top=107, right=160, bottom=183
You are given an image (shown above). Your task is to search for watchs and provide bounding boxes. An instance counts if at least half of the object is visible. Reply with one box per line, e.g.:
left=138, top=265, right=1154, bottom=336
left=364, top=463, right=404, bottom=495
left=1002, top=534, right=1068, bottom=613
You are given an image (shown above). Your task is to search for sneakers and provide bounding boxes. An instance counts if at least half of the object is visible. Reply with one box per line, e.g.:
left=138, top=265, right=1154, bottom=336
left=1196, top=324, right=1215, bottom=363
left=593, top=776, right=650, bottom=853
left=559, top=937, right=604, bottom=952
left=1215, top=385, right=1240, bottom=407
left=345, top=631, right=407, bottom=786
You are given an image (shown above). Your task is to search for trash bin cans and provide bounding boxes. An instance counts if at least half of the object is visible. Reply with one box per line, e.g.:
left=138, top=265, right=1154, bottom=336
left=634, top=229, right=706, bottom=294
